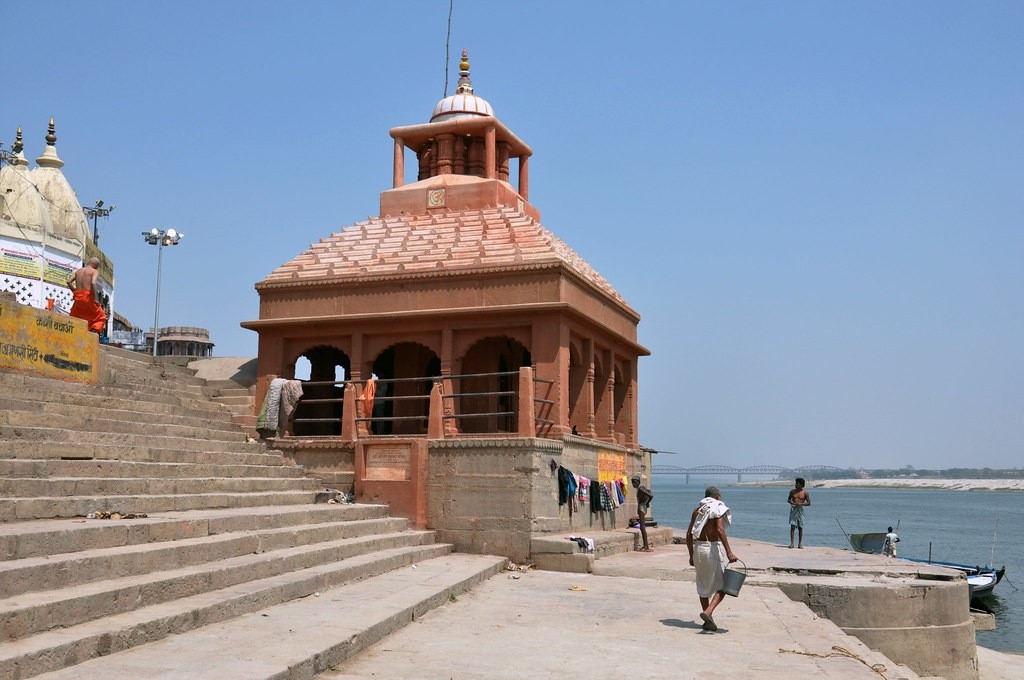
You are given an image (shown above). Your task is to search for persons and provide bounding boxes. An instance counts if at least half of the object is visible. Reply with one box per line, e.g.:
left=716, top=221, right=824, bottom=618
left=788, top=478, right=810, bottom=549
left=885, top=527, right=900, bottom=558
left=67, top=257, right=106, bottom=333
left=631, top=476, right=653, bottom=551
left=686, top=486, right=737, bottom=631
left=371, top=373, right=387, bottom=435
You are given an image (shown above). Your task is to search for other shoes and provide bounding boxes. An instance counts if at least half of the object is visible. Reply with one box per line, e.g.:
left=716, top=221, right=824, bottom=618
left=700, top=611, right=717, bottom=631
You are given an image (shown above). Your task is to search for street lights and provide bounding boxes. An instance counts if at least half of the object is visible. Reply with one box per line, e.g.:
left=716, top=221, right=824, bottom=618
left=0, top=142, right=23, bottom=170
left=142, top=227, right=184, bottom=357
left=83, top=200, right=116, bottom=247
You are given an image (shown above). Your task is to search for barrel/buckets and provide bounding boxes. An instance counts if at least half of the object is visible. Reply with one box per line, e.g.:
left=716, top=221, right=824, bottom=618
left=723, top=559, right=747, bottom=596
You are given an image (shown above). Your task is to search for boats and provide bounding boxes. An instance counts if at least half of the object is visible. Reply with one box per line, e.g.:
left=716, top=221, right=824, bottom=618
left=850, top=533, right=1005, bottom=612
